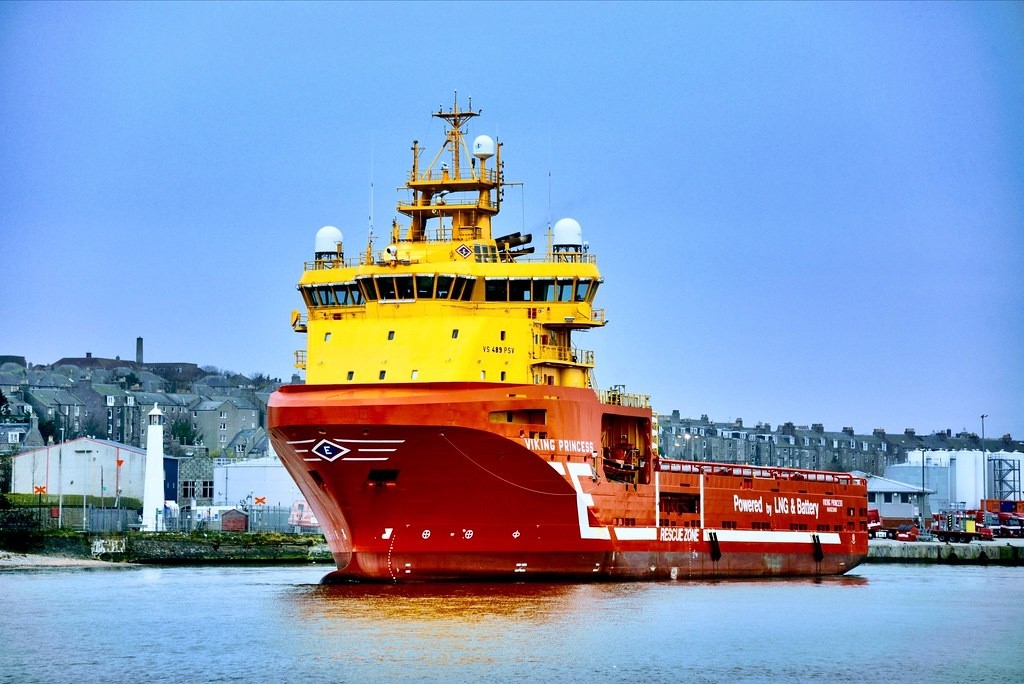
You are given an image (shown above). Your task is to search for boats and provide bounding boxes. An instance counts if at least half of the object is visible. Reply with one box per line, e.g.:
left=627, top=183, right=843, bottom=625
left=266, top=88, right=870, bottom=581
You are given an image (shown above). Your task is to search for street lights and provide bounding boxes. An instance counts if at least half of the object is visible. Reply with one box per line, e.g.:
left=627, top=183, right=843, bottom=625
left=980, top=414, right=988, bottom=529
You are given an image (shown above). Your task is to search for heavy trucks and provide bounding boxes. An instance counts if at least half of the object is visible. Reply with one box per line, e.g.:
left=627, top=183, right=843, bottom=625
left=929, top=509, right=1024, bottom=544
